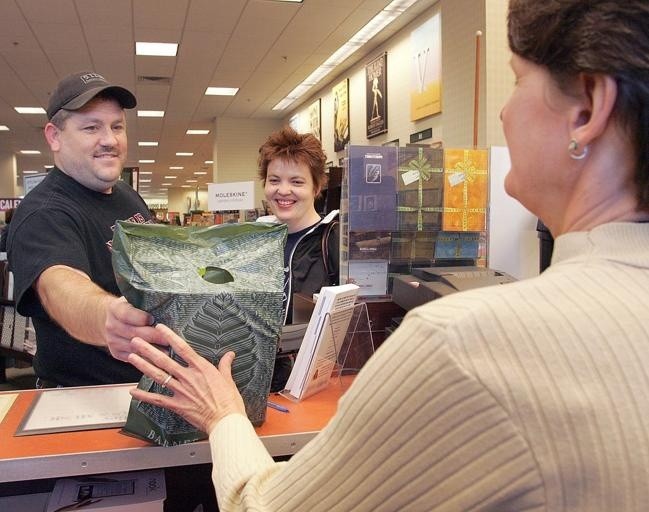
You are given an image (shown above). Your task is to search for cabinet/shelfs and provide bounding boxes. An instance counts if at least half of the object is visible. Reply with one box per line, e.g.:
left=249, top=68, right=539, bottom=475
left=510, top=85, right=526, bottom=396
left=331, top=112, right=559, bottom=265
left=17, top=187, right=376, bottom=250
left=314, top=165, right=343, bottom=218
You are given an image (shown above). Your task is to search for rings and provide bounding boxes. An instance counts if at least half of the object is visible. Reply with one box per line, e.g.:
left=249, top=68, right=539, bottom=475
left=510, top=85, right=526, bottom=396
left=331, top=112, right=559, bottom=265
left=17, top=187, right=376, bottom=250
left=162, top=376, right=172, bottom=389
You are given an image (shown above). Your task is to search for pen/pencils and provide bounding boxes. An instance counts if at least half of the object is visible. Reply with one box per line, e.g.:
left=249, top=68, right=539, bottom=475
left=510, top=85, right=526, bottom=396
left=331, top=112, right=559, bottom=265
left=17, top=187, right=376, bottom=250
left=267, top=401, right=289, bottom=413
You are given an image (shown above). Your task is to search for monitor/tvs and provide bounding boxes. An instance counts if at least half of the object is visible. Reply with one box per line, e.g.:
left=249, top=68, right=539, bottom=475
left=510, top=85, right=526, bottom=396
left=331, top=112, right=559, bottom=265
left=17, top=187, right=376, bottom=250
left=120, top=167, right=132, bottom=186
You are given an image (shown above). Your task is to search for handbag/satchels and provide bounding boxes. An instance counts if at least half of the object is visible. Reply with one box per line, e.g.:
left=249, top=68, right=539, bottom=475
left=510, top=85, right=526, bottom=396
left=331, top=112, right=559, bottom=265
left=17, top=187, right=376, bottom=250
left=107, top=216, right=289, bottom=450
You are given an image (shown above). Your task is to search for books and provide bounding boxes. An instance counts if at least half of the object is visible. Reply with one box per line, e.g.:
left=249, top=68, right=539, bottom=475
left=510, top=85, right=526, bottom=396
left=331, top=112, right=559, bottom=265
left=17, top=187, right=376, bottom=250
left=285, top=283, right=360, bottom=399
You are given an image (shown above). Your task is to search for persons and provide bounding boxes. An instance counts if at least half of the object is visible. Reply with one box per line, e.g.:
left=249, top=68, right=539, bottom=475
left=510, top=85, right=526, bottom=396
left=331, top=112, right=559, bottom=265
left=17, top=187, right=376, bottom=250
left=0, top=208, right=16, bottom=252
left=251, top=126, right=340, bottom=393
left=11, top=69, right=169, bottom=388
left=127, top=0, right=649, bottom=511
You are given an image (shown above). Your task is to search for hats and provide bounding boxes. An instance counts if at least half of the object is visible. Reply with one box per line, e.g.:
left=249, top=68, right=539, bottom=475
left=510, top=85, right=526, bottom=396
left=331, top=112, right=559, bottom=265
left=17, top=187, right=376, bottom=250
left=45, top=71, right=137, bottom=122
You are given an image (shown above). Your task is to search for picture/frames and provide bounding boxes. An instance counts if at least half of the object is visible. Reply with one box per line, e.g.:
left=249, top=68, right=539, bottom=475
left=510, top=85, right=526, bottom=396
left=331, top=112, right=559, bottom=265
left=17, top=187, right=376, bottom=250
left=365, top=51, right=389, bottom=141
